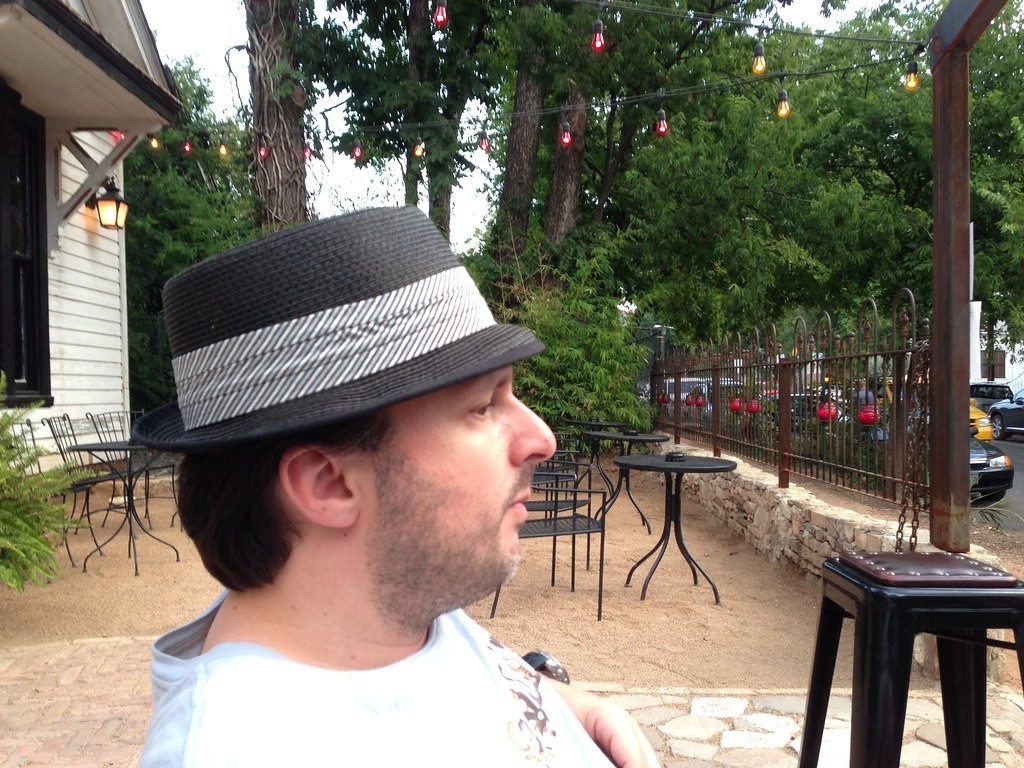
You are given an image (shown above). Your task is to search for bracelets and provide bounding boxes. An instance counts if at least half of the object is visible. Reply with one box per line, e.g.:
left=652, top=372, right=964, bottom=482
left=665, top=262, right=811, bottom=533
left=520, top=650, right=570, bottom=685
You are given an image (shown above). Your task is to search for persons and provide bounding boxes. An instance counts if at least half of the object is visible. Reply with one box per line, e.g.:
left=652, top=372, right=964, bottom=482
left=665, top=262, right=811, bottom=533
left=848, top=380, right=889, bottom=447
left=130, top=202, right=662, bottom=768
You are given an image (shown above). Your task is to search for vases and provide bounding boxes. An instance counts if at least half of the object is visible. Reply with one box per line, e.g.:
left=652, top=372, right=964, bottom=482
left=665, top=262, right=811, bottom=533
left=747, top=398, right=761, bottom=414
left=858, top=404, right=879, bottom=425
left=730, top=397, right=743, bottom=411
left=816, top=401, right=837, bottom=420
left=694, top=396, right=706, bottom=406
left=686, top=395, right=695, bottom=406
left=656, top=393, right=670, bottom=402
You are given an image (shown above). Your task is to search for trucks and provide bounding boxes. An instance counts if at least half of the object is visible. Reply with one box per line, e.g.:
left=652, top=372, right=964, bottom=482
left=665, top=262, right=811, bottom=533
left=876, top=377, right=994, bottom=442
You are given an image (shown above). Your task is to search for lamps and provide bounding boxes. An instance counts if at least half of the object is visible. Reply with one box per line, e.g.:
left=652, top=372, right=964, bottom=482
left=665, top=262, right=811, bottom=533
left=86, top=177, right=129, bottom=230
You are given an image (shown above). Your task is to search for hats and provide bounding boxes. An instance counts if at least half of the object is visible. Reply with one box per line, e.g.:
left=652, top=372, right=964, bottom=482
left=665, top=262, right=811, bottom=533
left=129, top=205, right=547, bottom=445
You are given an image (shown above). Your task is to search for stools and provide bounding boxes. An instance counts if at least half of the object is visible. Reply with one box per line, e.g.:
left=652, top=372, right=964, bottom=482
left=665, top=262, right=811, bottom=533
left=800, top=551, right=1024, bottom=768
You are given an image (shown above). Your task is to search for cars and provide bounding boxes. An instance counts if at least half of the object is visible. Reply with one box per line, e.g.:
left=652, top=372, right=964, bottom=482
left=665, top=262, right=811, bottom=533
left=987, top=388, right=1024, bottom=440
left=970, top=381, right=1014, bottom=412
left=869, top=410, right=1015, bottom=501
left=663, top=376, right=712, bottom=419
left=685, top=384, right=762, bottom=413
left=760, top=392, right=885, bottom=445
left=820, top=381, right=880, bottom=425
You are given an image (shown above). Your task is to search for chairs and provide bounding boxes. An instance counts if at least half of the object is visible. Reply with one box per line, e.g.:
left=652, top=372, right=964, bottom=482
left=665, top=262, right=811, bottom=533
left=12, top=409, right=178, bottom=566
left=490, top=429, right=607, bottom=622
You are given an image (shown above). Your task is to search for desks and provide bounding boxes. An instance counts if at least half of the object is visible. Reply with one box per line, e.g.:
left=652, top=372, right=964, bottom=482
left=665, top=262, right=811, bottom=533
left=583, top=431, right=670, bottom=534
left=613, top=455, right=737, bottom=605
left=68, top=442, right=180, bottom=577
left=562, top=418, right=633, bottom=499
